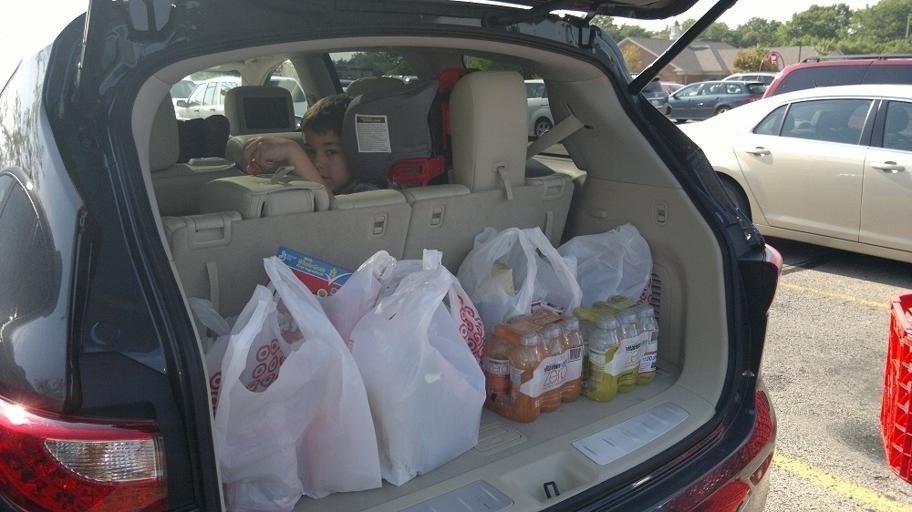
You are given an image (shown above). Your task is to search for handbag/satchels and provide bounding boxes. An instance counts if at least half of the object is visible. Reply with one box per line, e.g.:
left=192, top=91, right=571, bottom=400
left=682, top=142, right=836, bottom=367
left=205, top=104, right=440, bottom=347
left=185, top=255, right=384, bottom=512
left=350, top=267, right=489, bottom=487
left=456, top=222, right=657, bottom=316
left=316, top=250, right=487, bottom=364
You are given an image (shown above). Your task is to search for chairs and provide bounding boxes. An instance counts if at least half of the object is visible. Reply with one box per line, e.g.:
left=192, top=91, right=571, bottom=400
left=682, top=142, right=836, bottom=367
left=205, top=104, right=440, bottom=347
left=144, top=81, right=582, bottom=326
left=762, top=110, right=910, bottom=141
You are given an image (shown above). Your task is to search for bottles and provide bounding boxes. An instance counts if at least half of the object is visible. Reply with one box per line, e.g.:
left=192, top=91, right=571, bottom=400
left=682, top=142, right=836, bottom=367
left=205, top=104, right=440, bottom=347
left=482, top=294, right=660, bottom=423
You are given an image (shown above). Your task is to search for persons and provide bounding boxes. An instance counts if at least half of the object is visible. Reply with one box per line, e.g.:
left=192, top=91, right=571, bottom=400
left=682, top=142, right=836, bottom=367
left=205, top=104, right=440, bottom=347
left=239, top=91, right=383, bottom=197
